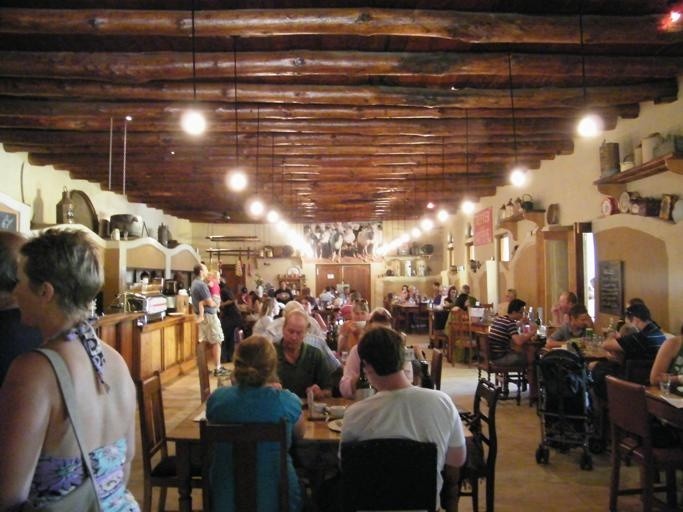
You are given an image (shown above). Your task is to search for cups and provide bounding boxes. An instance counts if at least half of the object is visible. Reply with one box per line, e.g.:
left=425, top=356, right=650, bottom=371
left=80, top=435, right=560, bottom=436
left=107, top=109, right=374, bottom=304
left=658, top=375, right=671, bottom=396
left=311, top=402, right=327, bottom=414
left=327, top=405, right=344, bottom=418
left=355, top=321, right=366, bottom=329
left=585, top=327, right=592, bottom=341
left=111, top=228, right=119, bottom=240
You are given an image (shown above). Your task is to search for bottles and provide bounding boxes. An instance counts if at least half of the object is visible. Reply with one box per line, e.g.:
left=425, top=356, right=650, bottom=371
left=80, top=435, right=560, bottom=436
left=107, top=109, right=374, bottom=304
left=157, top=224, right=168, bottom=247
left=335, top=351, right=348, bottom=363
left=498, top=197, right=521, bottom=218
left=521, top=306, right=541, bottom=326
left=55, top=192, right=73, bottom=225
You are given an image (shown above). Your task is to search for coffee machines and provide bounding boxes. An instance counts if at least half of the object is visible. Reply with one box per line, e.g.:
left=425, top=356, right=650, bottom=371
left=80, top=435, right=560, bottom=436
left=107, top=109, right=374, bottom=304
left=164, top=280, right=176, bottom=312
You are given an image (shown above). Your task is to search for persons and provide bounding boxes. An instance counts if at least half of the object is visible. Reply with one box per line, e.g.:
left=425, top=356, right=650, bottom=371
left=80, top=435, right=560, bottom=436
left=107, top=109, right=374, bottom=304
left=1, top=230, right=42, bottom=376
left=650, top=321, right=683, bottom=389
left=337, top=326, right=466, bottom=512
left=627, top=297, right=663, bottom=335
left=587, top=305, right=666, bottom=424
left=604, top=324, right=639, bottom=365
left=397, top=281, right=476, bottom=312
left=202, top=336, right=303, bottom=512
left=546, top=306, right=593, bottom=348
left=0, top=222, right=141, bottom=512
left=240, top=280, right=414, bottom=398
left=189, top=263, right=241, bottom=377
left=494, top=290, right=517, bottom=314
left=489, top=299, right=543, bottom=405
left=551, top=292, right=594, bottom=330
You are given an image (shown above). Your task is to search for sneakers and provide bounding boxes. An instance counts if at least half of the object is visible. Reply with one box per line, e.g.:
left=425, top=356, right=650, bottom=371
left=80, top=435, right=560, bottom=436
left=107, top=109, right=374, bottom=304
left=214, top=366, right=232, bottom=376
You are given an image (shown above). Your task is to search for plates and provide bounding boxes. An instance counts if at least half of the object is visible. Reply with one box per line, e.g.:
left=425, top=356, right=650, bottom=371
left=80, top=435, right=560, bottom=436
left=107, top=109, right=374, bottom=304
left=167, top=312, right=183, bottom=317
left=327, top=419, right=343, bottom=432
left=286, top=265, right=300, bottom=277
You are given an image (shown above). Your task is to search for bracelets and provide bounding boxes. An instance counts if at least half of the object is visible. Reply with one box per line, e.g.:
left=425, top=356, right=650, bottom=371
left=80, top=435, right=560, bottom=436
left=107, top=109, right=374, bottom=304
left=677, top=374, right=682, bottom=384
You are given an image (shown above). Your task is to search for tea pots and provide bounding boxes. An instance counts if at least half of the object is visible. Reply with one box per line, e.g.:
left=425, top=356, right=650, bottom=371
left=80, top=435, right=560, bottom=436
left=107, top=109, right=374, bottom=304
left=521, top=194, right=533, bottom=211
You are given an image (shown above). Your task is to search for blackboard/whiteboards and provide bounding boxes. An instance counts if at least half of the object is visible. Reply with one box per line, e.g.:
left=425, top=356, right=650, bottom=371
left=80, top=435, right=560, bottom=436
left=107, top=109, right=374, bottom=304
left=599, top=260, right=623, bottom=316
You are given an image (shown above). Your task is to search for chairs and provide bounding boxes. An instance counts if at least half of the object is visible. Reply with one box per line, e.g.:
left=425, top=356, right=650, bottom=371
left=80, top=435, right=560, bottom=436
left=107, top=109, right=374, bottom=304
left=131, top=292, right=683, bottom=511
